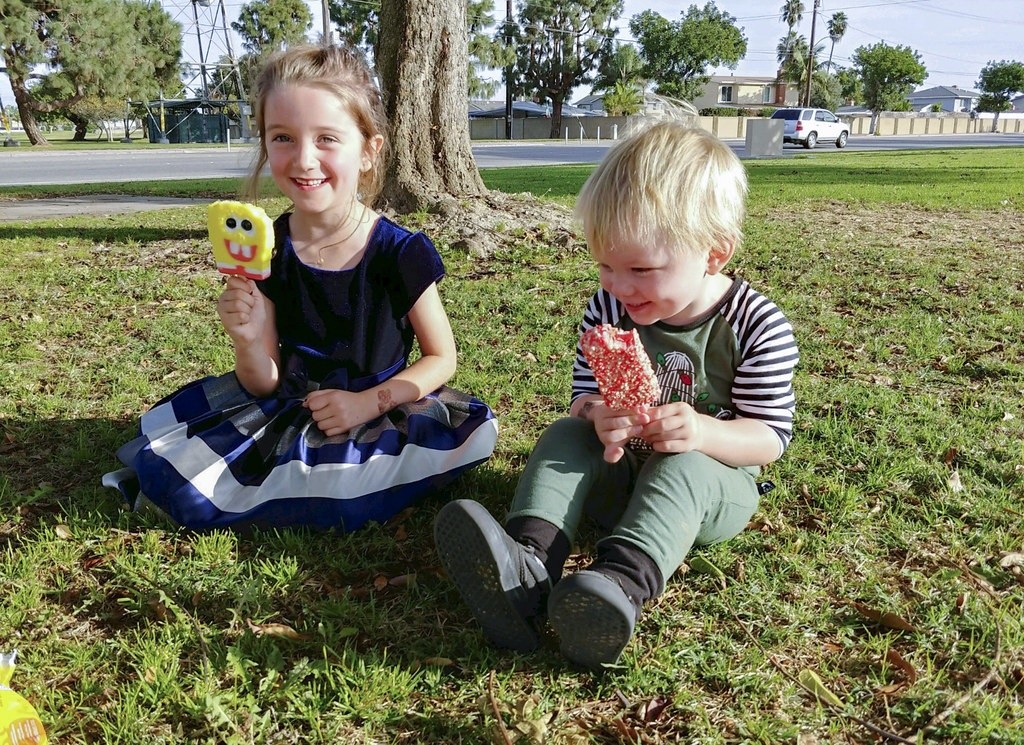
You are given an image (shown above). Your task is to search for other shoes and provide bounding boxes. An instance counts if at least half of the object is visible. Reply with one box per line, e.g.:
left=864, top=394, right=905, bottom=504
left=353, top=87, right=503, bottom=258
left=432, top=496, right=548, bottom=656
left=546, top=560, right=641, bottom=675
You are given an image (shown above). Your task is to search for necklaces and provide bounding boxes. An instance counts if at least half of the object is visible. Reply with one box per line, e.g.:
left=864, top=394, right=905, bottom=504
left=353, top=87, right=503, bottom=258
left=316, top=257, right=324, bottom=264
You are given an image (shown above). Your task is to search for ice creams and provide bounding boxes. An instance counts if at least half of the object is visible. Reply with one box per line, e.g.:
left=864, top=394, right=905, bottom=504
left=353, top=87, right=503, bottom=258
left=580, top=326, right=662, bottom=410
left=207, top=201, right=272, bottom=280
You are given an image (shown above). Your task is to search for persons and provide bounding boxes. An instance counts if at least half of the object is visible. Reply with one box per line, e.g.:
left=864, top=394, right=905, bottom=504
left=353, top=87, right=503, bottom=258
left=102, top=43, right=497, bottom=535
left=431, top=91, right=797, bottom=673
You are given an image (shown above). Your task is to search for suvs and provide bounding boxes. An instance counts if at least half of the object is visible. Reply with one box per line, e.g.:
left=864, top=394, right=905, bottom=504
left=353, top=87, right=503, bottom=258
left=770, top=106, right=849, bottom=149
